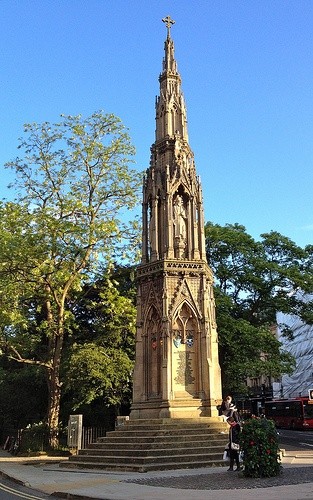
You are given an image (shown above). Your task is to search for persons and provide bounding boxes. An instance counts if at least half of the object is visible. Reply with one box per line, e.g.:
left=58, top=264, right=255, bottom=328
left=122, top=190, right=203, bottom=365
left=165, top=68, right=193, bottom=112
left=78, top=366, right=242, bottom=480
left=219, top=396, right=238, bottom=421
left=227, top=417, right=242, bottom=473
left=172, top=196, right=189, bottom=238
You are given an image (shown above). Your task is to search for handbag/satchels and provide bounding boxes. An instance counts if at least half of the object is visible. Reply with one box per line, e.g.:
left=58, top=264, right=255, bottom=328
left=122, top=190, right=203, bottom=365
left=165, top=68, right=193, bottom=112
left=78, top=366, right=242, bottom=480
left=223, top=443, right=230, bottom=462
left=230, top=443, right=239, bottom=450
left=237, top=449, right=244, bottom=463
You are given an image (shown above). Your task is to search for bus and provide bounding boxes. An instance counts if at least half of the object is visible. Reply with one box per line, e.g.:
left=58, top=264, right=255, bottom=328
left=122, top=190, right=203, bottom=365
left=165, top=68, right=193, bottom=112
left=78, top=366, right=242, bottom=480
left=264, top=396, right=313, bottom=431
